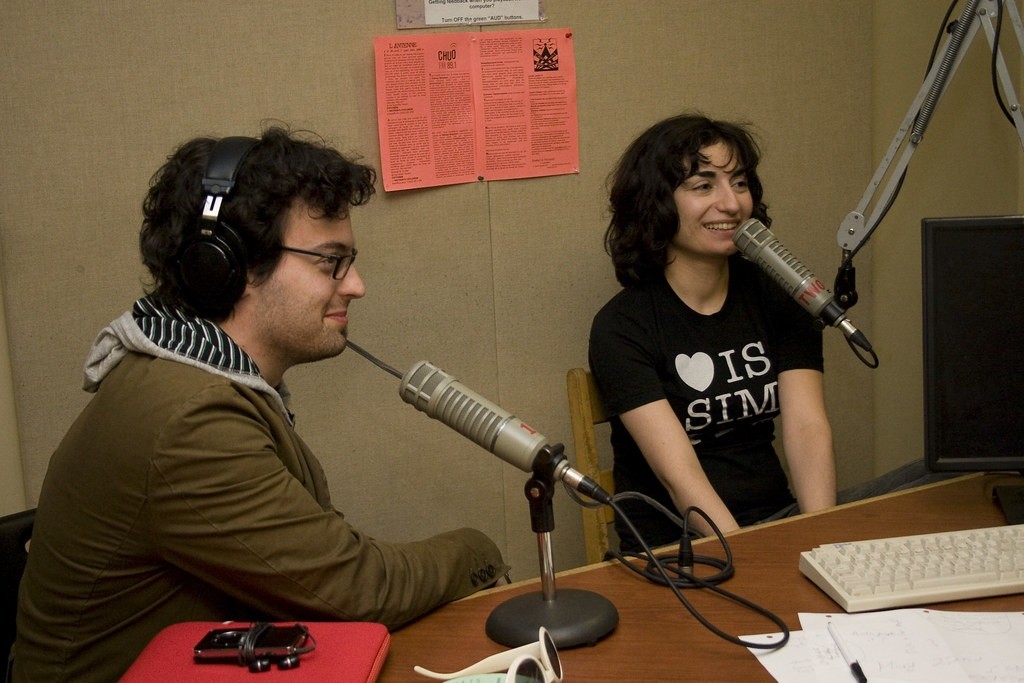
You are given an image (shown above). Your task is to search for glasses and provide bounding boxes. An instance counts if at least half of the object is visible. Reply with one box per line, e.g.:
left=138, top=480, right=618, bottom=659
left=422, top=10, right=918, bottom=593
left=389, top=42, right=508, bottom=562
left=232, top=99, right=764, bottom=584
left=282, top=245, right=358, bottom=280
left=413, top=626, right=564, bottom=683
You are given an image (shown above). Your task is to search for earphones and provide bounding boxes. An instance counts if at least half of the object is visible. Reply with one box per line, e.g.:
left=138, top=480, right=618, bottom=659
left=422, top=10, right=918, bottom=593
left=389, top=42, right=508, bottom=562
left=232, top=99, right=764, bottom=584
left=249, top=658, right=271, bottom=672
left=278, top=656, right=299, bottom=669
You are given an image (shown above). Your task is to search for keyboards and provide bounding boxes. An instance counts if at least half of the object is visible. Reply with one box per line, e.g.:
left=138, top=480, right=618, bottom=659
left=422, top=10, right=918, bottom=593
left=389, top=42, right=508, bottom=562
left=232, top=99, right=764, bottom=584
left=799, top=524, right=1024, bottom=616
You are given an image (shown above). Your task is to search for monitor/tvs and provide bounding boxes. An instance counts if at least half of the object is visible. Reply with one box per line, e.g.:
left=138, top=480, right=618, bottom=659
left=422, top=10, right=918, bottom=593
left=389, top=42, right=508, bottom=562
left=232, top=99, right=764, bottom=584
left=920, top=214, right=1024, bottom=527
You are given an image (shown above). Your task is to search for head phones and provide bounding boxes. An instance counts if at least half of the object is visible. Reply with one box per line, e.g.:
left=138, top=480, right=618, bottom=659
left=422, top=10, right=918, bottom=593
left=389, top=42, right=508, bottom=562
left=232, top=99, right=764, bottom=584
left=170, top=136, right=261, bottom=322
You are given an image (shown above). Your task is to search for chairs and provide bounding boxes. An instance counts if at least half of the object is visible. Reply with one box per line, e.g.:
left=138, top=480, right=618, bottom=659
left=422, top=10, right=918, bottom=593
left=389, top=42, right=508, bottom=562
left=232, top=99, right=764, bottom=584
left=0, top=507, right=36, bottom=683
left=565, top=369, right=622, bottom=568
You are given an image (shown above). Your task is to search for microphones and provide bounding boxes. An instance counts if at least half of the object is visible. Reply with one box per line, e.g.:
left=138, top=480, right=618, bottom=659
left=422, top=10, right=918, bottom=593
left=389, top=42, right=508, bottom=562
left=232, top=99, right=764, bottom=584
left=398, top=361, right=611, bottom=505
left=732, top=218, right=873, bottom=352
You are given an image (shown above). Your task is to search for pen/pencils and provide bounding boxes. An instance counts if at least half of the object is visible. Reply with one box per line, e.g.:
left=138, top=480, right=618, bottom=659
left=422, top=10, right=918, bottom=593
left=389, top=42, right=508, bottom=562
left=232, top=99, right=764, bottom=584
left=827, top=621, right=867, bottom=683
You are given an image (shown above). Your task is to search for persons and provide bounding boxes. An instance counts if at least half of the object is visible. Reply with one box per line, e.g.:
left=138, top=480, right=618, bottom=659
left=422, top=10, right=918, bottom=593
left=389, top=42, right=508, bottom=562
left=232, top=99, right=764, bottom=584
left=587, top=116, right=839, bottom=560
left=8, top=128, right=512, bottom=683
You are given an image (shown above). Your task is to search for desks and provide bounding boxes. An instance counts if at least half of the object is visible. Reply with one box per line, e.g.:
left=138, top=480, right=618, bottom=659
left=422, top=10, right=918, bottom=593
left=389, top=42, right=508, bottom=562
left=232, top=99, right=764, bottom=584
left=373, top=471, right=1024, bottom=683
